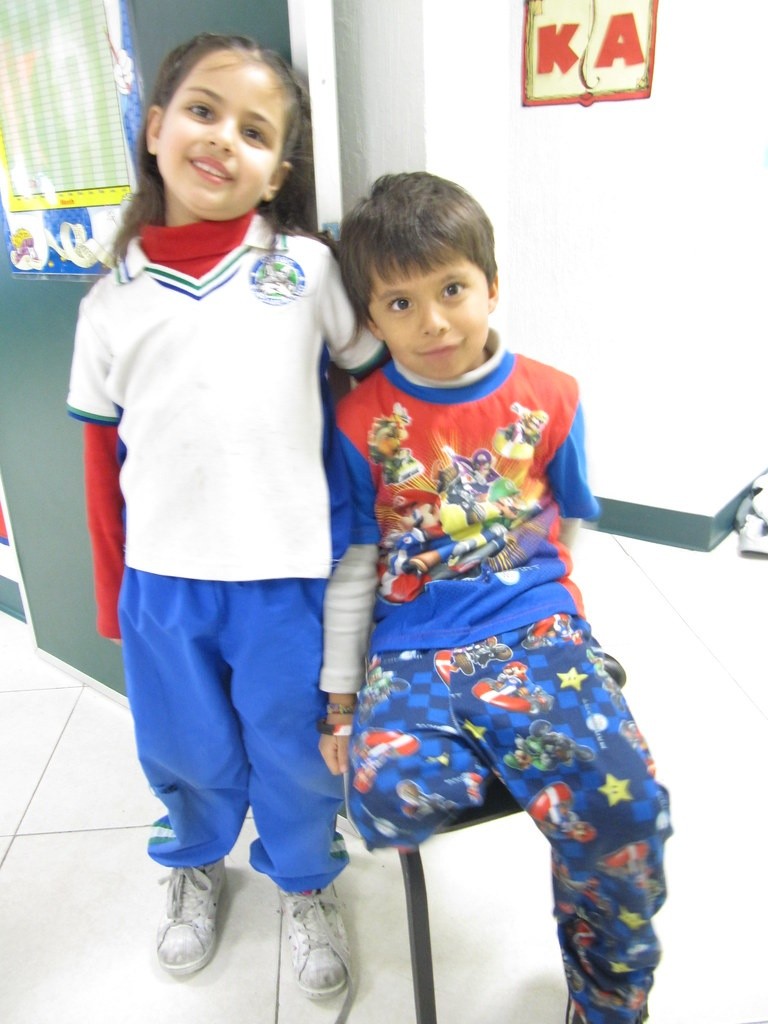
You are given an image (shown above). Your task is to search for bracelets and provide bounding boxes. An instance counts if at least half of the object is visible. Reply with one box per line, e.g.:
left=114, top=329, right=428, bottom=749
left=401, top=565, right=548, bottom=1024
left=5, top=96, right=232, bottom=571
left=324, top=703, right=355, bottom=715
left=317, top=720, right=352, bottom=737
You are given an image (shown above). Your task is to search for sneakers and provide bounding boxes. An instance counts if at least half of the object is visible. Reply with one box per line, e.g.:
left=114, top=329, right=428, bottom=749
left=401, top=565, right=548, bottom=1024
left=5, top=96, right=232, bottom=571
left=276, top=878, right=350, bottom=998
left=156, top=855, right=227, bottom=977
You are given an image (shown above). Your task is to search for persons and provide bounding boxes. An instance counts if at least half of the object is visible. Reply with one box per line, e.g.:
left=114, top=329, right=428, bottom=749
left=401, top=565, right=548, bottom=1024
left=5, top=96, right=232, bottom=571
left=64, top=29, right=398, bottom=1024
left=318, top=172, right=674, bottom=1023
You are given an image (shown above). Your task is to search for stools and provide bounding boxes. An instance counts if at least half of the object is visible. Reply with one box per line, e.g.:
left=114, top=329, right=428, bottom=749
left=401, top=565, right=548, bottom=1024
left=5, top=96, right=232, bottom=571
left=334, top=797, right=528, bottom=1024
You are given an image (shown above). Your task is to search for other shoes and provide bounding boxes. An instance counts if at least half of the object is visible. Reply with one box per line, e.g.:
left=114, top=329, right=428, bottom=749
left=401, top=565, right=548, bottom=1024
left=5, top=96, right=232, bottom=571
left=594, top=651, right=626, bottom=689
